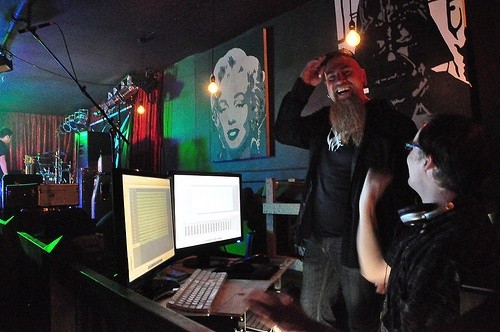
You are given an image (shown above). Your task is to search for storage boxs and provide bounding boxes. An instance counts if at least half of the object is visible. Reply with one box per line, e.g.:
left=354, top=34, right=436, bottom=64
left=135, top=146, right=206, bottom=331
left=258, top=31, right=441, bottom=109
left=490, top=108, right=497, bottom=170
left=37, top=183, right=80, bottom=206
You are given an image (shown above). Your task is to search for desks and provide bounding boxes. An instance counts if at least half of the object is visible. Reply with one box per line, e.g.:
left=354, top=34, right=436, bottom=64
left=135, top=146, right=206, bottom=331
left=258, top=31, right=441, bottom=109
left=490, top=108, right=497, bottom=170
left=73, top=248, right=500, bottom=332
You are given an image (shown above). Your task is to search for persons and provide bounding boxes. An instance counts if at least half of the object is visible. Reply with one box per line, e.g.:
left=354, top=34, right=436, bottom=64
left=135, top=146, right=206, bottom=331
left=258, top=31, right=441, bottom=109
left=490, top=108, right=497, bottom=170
left=0, top=127, right=14, bottom=176
left=274, top=48, right=419, bottom=332
left=382, top=108, right=500, bottom=332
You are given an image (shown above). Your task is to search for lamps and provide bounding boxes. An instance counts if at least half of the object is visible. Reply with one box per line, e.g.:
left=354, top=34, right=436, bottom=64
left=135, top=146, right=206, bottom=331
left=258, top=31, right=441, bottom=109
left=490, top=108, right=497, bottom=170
left=208, top=75, right=219, bottom=94
left=0, top=54, right=13, bottom=73
left=345, top=19, right=360, bottom=47
left=137, top=99, right=146, bottom=114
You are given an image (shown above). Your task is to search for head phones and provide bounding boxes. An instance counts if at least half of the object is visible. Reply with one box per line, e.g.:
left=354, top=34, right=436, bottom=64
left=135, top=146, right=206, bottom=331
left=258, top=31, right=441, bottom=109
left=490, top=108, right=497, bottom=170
left=397, top=197, right=461, bottom=227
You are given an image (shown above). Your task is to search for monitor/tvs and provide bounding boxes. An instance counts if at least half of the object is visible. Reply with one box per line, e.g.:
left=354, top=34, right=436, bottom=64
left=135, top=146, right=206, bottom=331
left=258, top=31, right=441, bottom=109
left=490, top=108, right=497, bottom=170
left=112, top=168, right=176, bottom=288
left=168, top=170, right=243, bottom=258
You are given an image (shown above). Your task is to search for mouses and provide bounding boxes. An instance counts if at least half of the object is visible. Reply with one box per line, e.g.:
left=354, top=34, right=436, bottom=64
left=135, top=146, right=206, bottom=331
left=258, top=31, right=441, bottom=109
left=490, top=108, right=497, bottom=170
left=230, top=262, right=255, bottom=273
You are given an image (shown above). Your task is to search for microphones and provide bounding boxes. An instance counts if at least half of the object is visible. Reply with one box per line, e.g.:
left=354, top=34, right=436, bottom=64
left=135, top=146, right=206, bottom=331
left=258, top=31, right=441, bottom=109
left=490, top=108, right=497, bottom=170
left=18, top=21, right=53, bottom=34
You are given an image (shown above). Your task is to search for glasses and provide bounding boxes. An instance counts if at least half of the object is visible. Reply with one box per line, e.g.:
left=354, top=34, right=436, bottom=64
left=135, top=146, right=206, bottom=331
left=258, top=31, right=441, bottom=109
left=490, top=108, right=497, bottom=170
left=320, top=48, right=369, bottom=68
left=404, top=138, right=424, bottom=151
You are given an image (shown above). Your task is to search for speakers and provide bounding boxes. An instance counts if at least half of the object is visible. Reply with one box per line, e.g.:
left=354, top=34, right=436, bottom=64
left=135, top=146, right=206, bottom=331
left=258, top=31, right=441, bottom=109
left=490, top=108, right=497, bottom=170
left=75, top=130, right=111, bottom=184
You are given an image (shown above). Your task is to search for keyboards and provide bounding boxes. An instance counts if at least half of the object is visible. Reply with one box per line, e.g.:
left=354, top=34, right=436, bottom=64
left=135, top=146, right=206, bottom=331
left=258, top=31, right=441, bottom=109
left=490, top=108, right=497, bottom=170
left=166, top=268, right=228, bottom=316
left=237, top=311, right=273, bottom=332
